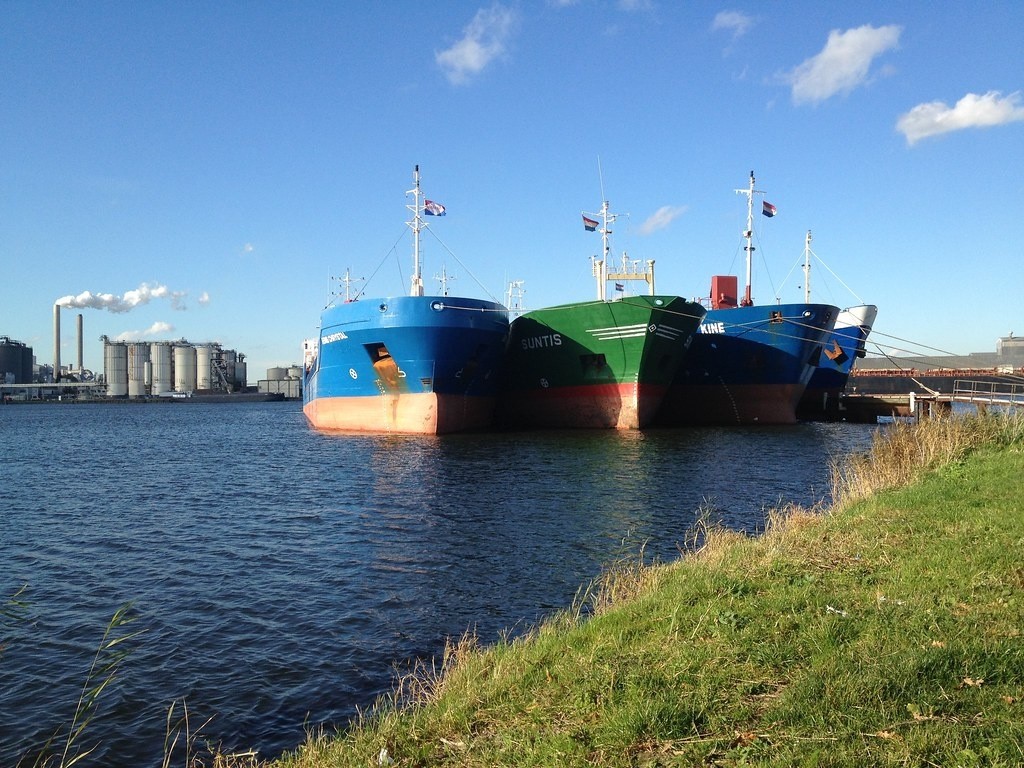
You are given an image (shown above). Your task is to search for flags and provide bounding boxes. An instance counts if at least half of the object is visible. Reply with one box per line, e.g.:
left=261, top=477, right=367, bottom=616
left=762, top=201, right=777, bottom=218
left=424, top=200, right=446, bottom=217
left=615, top=283, right=624, bottom=292
left=583, top=216, right=599, bottom=232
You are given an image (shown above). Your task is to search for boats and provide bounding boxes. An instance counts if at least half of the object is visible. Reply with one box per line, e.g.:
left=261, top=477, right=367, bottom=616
left=661, top=170, right=840, bottom=425
left=301, top=164, right=509, bottom=436
left=769, top=228, right=881, bottom=423
left=503, top=202, right=709, bottom=429
